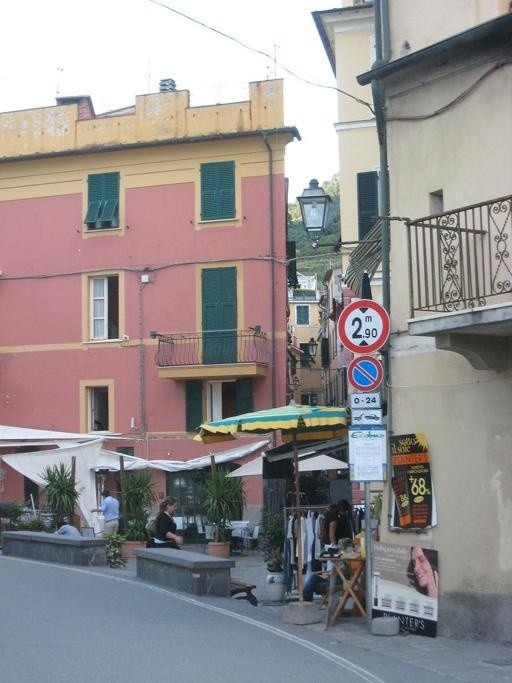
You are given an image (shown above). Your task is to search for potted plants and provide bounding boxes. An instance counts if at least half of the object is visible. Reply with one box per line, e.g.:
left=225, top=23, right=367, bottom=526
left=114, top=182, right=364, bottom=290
left=260, top=510, right=287, bottom=606
left=201, top=465, right=247, bottom=559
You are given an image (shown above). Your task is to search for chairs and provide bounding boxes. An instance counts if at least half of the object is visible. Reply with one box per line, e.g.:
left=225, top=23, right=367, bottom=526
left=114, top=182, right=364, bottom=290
left=172, top=514, right=261, bottom=554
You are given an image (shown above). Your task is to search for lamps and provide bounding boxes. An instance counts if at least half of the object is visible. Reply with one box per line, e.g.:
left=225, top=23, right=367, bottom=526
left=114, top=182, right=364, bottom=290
left=290, top=336, right=318, bottom=365
left=297, top=176, right=381, bottom=249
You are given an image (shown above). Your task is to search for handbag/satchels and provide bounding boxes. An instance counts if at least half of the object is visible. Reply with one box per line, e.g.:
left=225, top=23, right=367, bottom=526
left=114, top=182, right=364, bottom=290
left=145, top=520, right=168, bottom=542
left=318, top=516, right=346, bottom=541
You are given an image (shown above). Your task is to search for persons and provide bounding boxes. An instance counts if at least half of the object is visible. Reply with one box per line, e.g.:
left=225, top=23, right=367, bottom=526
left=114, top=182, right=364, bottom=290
left=151, top=495, right=184, bottom=549
left=90, top=490, right=121, bottom=535
left=323, top=499, right=353, bottom=570
left=409, top=546, right=438, bottom=600
left=55, top=522, right=82, bottom=536
left=301, top=536, right=355, bottom=600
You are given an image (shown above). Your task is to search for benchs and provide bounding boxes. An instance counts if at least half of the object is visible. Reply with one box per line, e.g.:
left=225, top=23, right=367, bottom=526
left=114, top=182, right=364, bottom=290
left=231, top=579, right=259, bottom=607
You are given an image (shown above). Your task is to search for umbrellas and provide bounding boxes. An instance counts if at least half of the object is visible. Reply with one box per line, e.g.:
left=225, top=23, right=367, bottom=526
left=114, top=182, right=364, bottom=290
left=193, top=399, right=352, bottom=602
left=225, top=449, right=349, bottom=574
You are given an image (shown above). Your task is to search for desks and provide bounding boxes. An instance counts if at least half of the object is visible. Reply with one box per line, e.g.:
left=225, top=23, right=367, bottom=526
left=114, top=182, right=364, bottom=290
left=319, top=549, right=367, bottom=631
